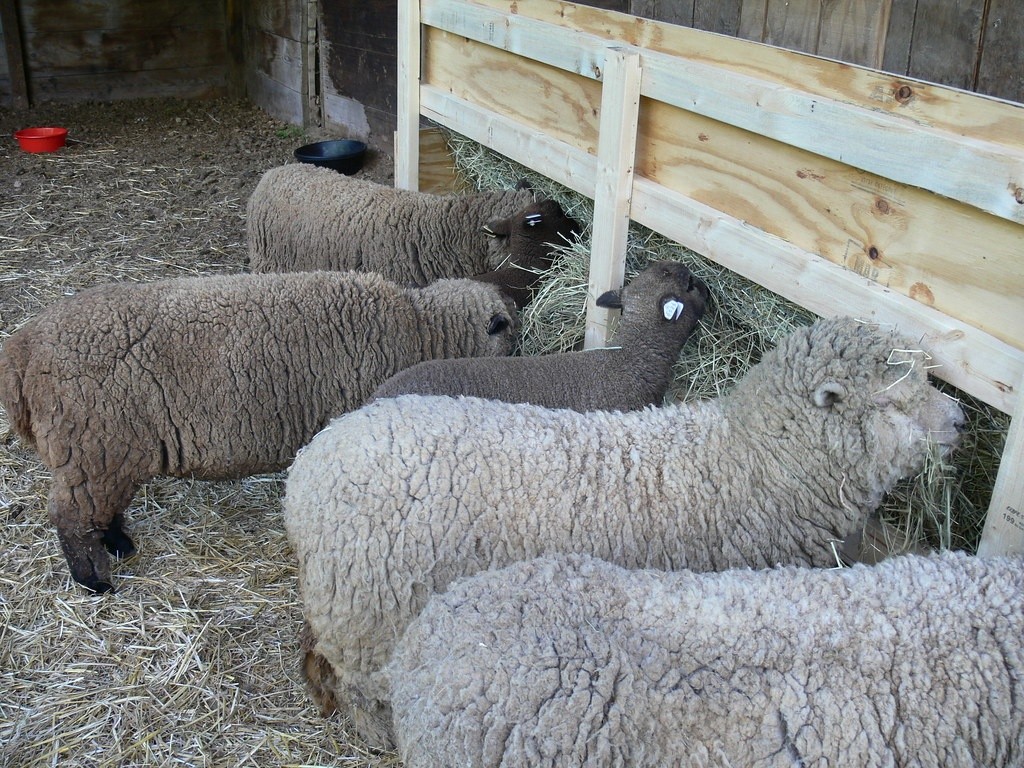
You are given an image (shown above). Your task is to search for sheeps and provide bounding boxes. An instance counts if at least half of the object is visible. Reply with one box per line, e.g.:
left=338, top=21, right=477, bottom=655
left=244, top=161, right=591, bottom=310
left=2, top=268, right=525, bottom=601
left=371, top=263, right=713, bottom=411
left=275, top=313, right=967, bottom=713
left=387, top=549, right=1024, bottom=767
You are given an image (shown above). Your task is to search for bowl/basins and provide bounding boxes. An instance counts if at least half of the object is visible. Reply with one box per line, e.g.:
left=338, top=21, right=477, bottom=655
left=14, top=127, right=69, bottom=152
left=294, top=140, right=367, bottom=176
